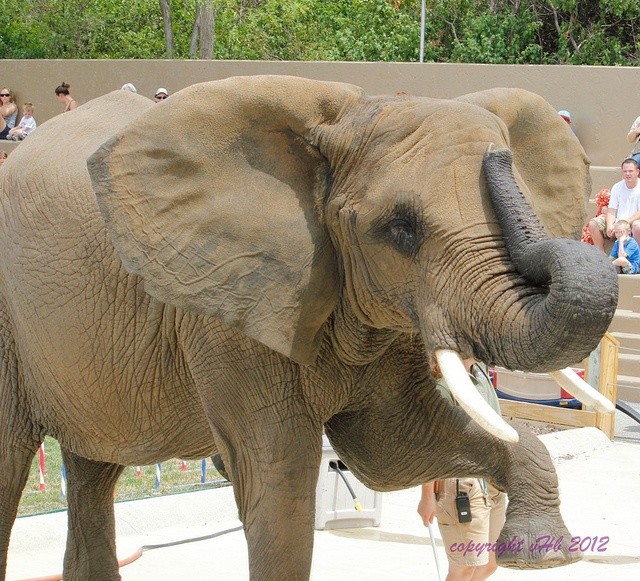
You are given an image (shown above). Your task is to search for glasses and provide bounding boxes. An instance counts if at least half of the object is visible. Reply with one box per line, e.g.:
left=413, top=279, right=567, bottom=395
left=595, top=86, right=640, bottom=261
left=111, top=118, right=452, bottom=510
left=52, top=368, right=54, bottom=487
left=0, top=94, right=9, bottom=97
left=156, top=97, right=166, bottom=99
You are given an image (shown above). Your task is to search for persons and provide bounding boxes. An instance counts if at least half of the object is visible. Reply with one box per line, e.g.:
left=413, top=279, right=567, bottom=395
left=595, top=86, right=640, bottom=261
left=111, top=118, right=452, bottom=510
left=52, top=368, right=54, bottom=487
left=627, top=115, right=640, bottom=159
left=0, top=87, right=18, bottom=140
left=6, top=102, right=37, bottom=140
left=557, top=110, right=571, bottom=125
left=603, top=221, right=640, bottom=275
left=121, top=83, right=137, bottom=94
left=417, top=357, right=507, bottom=581
left=54, top=81, right=77, bottom=113
left=0, top=151, right=7, bottom=165
left=154, top=88, right=169, bottom=103
left=589, top=159, right=639, bottom=251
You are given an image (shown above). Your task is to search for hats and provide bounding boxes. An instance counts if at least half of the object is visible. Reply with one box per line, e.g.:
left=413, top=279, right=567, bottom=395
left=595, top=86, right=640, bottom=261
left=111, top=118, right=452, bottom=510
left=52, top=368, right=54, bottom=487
left=558, top=111, right=570, bottom=120
left=156, top=88, right=167, bottom=95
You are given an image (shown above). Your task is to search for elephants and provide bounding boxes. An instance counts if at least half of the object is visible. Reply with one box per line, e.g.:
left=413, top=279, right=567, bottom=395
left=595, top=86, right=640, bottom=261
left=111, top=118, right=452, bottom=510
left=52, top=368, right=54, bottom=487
left=0, top=74, right=619, bottom=580
